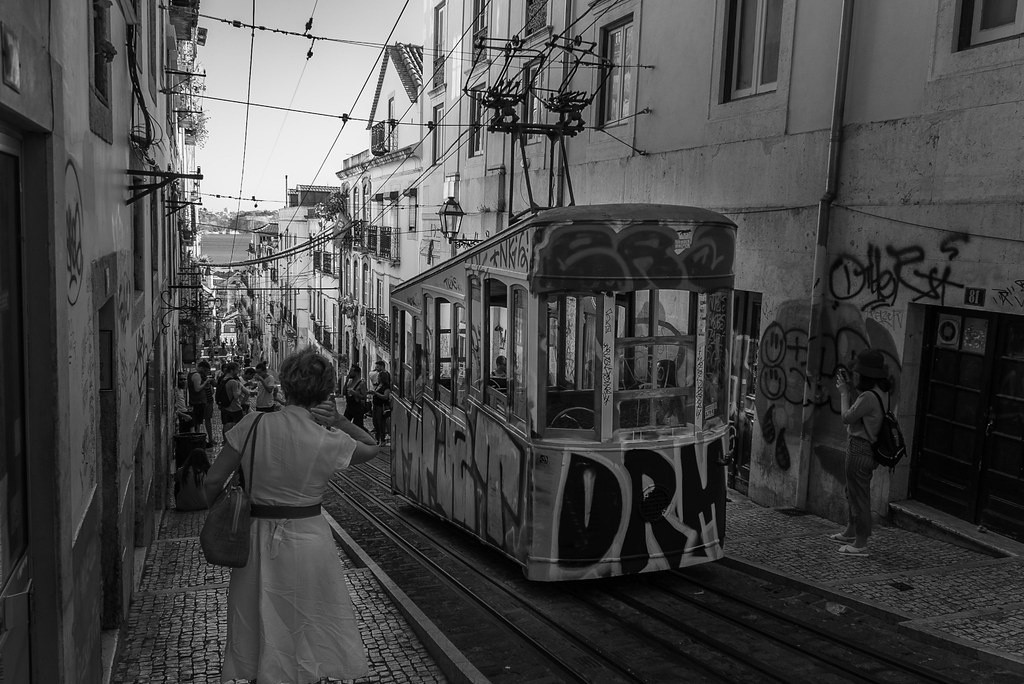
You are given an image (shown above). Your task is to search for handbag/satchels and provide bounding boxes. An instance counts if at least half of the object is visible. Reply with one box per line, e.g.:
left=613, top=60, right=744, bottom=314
left=350, top=397, right=372, bottom=416
left=200, top=410, right=267, bottom=568
left=383, top=409, right=391, bottom=417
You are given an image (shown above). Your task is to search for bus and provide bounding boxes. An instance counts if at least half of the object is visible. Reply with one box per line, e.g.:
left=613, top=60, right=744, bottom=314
left=389, top=203, right=739, bottom=582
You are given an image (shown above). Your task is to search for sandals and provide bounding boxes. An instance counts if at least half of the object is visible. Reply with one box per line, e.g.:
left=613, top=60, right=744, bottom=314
left=827, top=532, right=856, bottom=545
left=835, top=545, right=870, bottom=557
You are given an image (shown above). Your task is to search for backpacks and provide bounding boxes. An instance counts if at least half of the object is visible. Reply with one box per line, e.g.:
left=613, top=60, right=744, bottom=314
left=861, top=389, right=908, bottom=474
left=215, top=375, right=235, bottom=408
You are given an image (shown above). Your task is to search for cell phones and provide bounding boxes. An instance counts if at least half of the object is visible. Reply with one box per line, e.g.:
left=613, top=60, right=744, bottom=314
left=839, top=369, right=847, bottom=381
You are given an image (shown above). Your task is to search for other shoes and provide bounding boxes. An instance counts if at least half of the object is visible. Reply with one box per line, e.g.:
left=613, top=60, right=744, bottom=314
left=371, top=429, right=391, bottom=447
left=210, top=440, right=217, bottom=444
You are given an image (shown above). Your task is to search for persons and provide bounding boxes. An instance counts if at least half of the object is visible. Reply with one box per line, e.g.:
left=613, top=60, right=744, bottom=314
left=343, top=361, right=391, bottom=447
left=491, top=356, right=507, bottom=377
left=205, top=346, right=379, bottom=684
left=830, top=350, right=909, bottom=557
left=174, top=338, right=275, bottom=445
left=173, top=448, right=211, bottom=511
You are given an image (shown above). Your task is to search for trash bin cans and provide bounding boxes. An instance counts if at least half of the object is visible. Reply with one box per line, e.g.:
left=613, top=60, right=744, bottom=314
left=174, top=432, right=208, bottom=471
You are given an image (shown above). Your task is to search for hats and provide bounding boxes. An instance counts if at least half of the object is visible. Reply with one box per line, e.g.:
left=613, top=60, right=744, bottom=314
left=857, top=348, right=889, bottom=379
left=373, top=367, right=377, bottom=371
